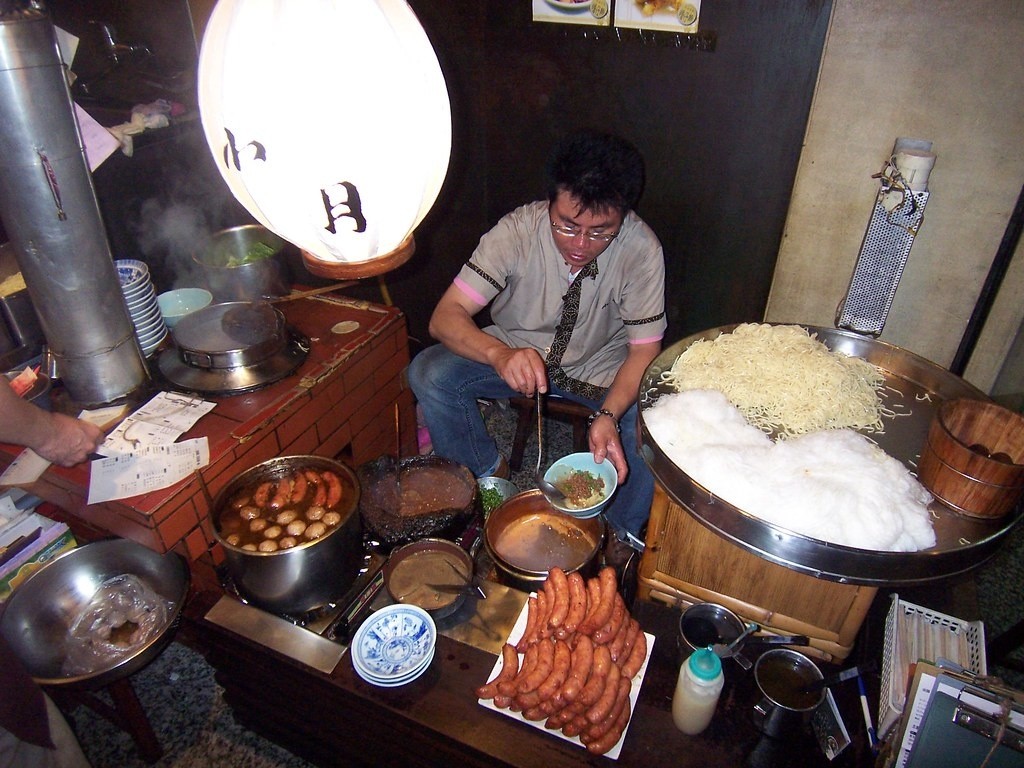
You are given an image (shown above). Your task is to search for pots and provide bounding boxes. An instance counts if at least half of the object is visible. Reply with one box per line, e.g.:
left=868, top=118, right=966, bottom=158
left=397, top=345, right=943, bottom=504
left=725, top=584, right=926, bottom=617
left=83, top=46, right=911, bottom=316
left=357, top=453, right=479, bottom=542
left=191, top=224, right=296, bottom=304
left=209, top=454, right=361, bottom=611
left=484, top=488, right=606, bottom=580
left=384, top=538, right=483, bottom=620
left=173, top=302, right=286, bottom=371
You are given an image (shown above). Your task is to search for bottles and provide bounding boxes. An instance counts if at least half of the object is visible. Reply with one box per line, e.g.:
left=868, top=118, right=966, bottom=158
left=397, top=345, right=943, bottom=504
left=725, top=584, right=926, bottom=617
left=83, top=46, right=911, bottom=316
left=672, top=644, right=724, bottom=735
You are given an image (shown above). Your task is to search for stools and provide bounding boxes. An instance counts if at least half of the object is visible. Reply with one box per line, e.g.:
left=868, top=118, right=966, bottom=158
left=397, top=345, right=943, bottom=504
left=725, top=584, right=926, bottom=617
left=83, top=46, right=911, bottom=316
left=507, top=394, right=595, bottom=471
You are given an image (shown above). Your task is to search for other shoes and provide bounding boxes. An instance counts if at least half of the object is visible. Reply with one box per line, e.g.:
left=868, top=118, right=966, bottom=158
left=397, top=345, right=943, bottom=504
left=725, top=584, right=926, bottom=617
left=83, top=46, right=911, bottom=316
left=491, top=453, right=510, bottom=479
left=603, top=528, right=635, bottom=577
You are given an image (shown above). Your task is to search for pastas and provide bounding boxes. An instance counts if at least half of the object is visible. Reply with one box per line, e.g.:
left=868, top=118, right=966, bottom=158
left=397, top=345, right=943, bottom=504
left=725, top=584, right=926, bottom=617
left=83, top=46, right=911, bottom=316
left=643, top=323, right=934, bottom=448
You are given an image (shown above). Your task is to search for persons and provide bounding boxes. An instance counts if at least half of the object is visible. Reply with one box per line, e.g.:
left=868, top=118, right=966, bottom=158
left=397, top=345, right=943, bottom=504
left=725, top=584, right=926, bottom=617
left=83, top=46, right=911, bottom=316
left=0, top=374, right=105, bottom=469
left=407, top=128, right=667, bottom=581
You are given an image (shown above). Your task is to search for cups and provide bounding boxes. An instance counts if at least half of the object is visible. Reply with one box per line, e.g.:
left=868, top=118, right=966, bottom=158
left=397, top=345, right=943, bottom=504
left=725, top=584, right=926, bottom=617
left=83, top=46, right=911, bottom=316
left=677, top=603, right=753, bottom=674
left=754, top=648, right=826, bottom=737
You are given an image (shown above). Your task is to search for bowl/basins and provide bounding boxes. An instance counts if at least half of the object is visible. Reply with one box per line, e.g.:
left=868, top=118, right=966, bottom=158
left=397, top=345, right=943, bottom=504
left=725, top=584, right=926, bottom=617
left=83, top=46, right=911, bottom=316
left=0, top=535, right=203, bottom=694
left=543, top=452, right=618, bottom=519
left=114, top=259, right=214, bottom=358
left=351, top=603, right=437, bottom=687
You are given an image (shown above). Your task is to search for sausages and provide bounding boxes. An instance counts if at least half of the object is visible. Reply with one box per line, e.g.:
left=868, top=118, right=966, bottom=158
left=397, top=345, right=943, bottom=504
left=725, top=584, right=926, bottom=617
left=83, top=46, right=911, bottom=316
left=477, top=564, right=648, bottom=754
left=254, top=470, right=343, bottom=508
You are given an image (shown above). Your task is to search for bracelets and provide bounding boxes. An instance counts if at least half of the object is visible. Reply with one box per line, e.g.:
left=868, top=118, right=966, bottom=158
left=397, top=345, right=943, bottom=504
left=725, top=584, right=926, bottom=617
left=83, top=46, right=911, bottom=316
left=587, top=409, right=621, bottom=433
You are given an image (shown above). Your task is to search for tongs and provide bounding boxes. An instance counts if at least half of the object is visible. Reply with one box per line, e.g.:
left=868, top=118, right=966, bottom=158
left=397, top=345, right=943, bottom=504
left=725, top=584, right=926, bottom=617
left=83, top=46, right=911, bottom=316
left=423, top=560, right=486, bottom=599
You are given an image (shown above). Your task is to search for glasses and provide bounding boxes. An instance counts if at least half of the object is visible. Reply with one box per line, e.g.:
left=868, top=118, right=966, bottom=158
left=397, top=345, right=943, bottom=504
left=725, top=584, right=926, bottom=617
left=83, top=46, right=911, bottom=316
left=548, top=205, right=622, bottom=242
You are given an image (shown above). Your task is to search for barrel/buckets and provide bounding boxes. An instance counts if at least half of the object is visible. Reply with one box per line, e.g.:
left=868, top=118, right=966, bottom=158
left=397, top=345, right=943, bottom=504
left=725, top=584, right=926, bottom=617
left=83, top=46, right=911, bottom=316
left=918, top=401, right=1024, bottom=518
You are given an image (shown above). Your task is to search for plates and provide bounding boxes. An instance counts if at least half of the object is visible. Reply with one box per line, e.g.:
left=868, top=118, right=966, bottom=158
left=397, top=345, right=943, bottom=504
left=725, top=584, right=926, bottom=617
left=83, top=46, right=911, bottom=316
left=477, top=592, right=655, bottom=759
left=4, top=371, right=50, bottom=401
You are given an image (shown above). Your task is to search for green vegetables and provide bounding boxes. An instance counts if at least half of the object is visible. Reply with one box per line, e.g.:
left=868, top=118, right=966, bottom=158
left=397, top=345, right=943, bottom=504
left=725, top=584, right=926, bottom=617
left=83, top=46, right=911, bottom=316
left=227, top=242, right=275, bottom=267
left=480, top=483, right=504, bottom=516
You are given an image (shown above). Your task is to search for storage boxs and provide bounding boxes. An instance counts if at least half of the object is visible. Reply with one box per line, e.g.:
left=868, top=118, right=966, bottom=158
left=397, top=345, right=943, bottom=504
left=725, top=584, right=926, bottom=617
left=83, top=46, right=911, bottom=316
left=876, top=593, right=988, bottom=739
